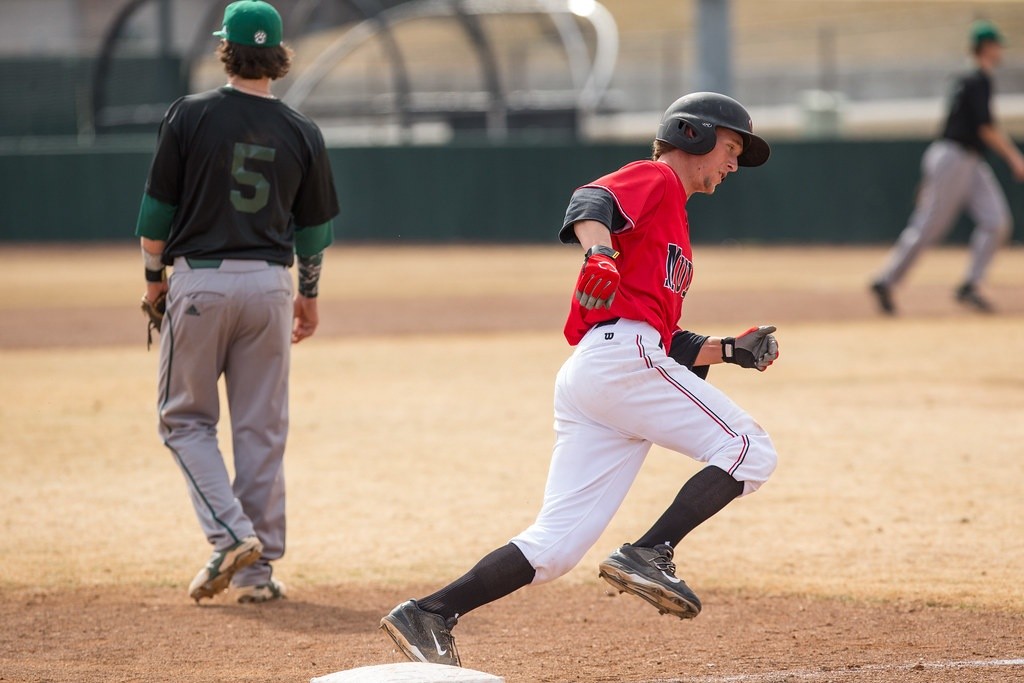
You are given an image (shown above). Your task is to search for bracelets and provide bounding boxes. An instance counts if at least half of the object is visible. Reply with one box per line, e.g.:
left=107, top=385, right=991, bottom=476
left=140, top=249, right=167, bottom=283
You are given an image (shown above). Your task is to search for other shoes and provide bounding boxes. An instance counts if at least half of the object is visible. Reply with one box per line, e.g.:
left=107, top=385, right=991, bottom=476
left=954, top=282, right=993, bottom=314
left=869, top=276, right=895, bottom=314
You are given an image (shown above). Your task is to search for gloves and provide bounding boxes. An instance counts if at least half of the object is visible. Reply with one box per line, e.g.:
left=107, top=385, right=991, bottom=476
left=719, top=325, right=779, bottom=372
left=576, top=245, right=620, bottom=309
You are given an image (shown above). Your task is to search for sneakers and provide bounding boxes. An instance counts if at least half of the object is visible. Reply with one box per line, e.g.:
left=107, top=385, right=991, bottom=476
left=235, top=582, right=284, bottom=604
left=598, top=542, right=700, bottom=621
left=376, top=599, right=463, bottom=668
left=189, top=538, right=264, bottom=609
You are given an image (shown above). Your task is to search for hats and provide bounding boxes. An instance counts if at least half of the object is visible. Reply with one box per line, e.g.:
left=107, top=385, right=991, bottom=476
left=211, top=1, right=283, bottom=47
left=969, top=21, right=1006, bottom=52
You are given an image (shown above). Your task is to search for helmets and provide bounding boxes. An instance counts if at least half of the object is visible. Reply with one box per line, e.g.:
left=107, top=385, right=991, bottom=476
left=656, top=92, right=771, bottom=168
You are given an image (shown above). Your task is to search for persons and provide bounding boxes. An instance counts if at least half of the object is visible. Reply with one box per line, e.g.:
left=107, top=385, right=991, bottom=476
left=868, top=19, right=1024, bottom=313
left=378, top=95, right=780, bottom=671
left=138, top=0, right=344, bottom=607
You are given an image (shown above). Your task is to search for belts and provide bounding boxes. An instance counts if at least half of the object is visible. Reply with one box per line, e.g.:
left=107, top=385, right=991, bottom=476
left=183, top=259, right=284, bottom=268
left=594, top=318, right=665, bottom=349
